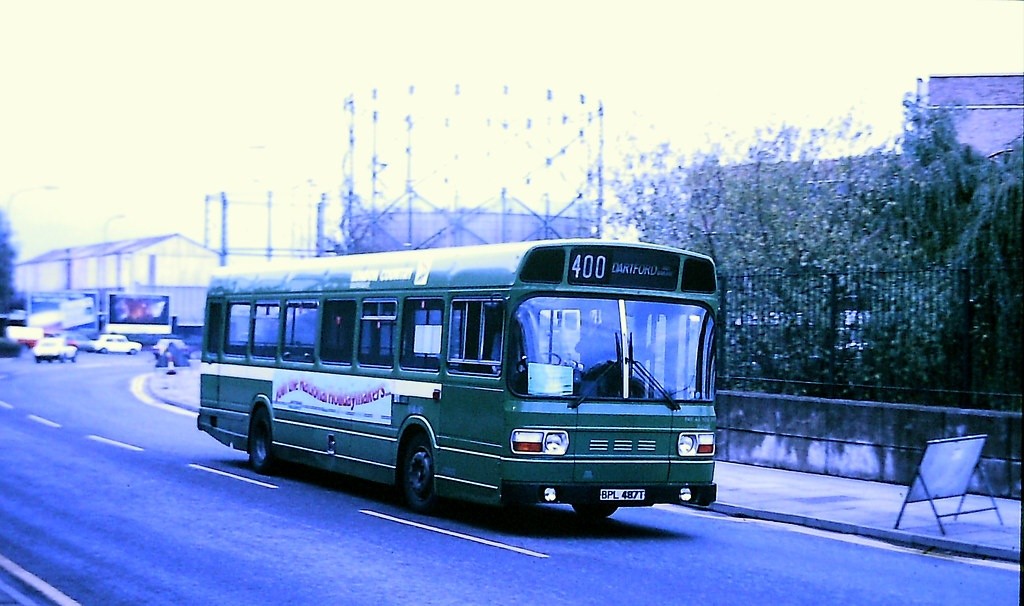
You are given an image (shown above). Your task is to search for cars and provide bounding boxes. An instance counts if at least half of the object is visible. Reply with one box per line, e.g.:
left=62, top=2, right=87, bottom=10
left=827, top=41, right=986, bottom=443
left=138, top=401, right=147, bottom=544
left=152, top=339, right=190, bottom=358
left=59, top=330, right=95, bottom=352
left=31, top=338, right=80, bottom=364
left=92, top=333, right=144, bottom=356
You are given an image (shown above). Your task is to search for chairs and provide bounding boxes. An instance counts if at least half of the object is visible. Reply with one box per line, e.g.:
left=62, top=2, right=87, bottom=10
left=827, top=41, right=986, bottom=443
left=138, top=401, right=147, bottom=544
left=230, top=341, right=442, bottom=370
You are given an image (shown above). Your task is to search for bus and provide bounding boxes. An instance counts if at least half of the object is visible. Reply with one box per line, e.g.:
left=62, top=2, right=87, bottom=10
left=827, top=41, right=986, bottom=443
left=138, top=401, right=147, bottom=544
left=196, top=237, right=722, bottom=521
